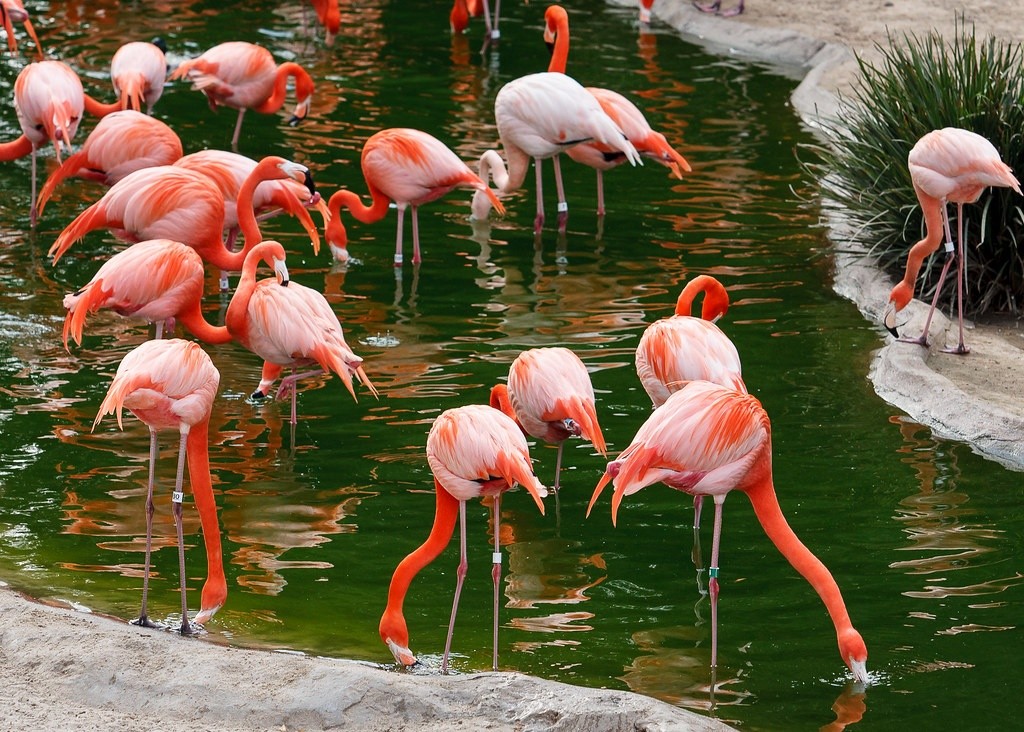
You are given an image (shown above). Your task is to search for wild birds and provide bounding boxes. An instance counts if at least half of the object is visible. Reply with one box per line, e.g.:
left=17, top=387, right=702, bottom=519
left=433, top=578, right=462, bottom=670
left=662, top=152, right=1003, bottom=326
left=323, top=128, right=506, bottom=267
left=453, top=1, right=643, bottom=233
left=490, top=347, right=607, bottom=492
left=544, top=6, right=693, bottom=215
left=1, top=1, right=343, bottom=273
left=883, top=127, right=1023, bottom=355
left=635, top=274, right=750, bottom=526
left=585, top=379, right=870, bottom=689
left=379, top=405, right=550, bottom=672
left=224, top=241, right=380, bottom=425
left=91, top=337, right=229, bottom=641
left=64, top=239, right=232, bottom=357
